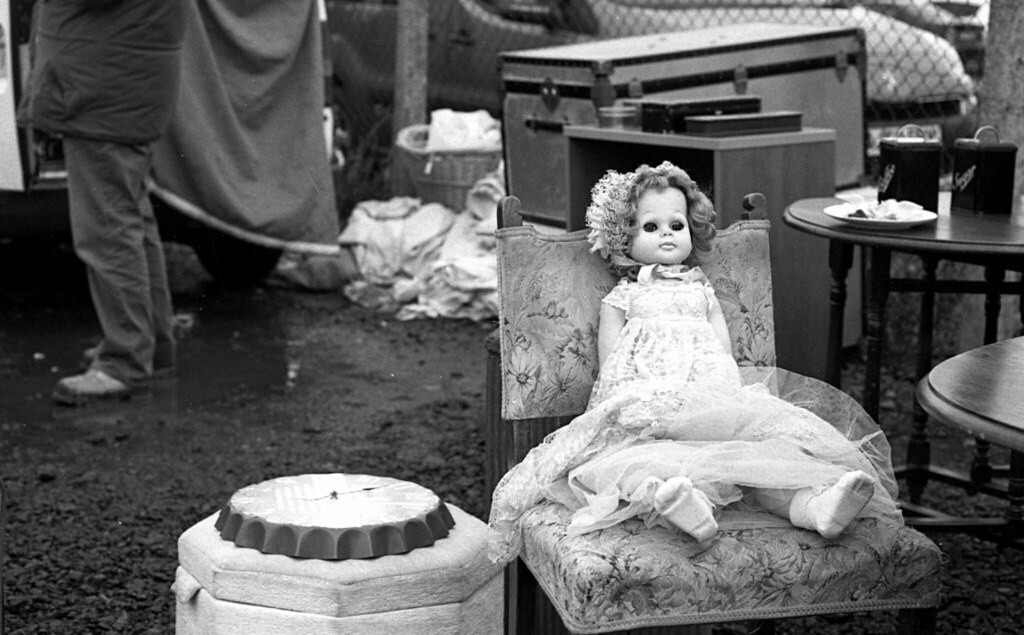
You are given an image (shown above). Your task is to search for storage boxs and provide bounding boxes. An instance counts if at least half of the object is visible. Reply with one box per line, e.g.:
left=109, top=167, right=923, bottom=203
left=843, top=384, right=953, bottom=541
left=499, top=18, right=868, bottom=221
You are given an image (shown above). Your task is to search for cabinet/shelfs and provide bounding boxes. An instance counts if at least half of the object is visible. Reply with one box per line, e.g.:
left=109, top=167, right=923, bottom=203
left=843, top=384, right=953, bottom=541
left=564, top=126, right=839, bottom=382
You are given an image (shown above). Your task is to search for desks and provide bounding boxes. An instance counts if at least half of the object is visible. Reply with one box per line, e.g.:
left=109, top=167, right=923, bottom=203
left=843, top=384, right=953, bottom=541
left=899, top=332, right=1024, bottom=555
left=786, top=196, right=1024, bottom=505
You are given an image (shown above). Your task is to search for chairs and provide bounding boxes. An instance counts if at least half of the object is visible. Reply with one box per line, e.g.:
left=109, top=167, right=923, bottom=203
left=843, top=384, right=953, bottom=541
left=483, top=194, right=944, bottom=635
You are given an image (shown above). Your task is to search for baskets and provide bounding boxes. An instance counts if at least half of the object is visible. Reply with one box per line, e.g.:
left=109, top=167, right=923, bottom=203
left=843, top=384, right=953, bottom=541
left=397, top=122, right=505, bottom=215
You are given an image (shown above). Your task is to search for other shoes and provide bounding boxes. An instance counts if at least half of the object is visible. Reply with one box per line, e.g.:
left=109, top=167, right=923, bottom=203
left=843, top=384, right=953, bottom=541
left=172, top=278, right=267, bottom=318
left=80, top=347, right=175, bottom=371
left=52, top=366, right=154, bottom=405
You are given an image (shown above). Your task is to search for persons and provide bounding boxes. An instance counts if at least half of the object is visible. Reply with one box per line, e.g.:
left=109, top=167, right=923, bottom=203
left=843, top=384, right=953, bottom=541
left=148, top=0, right=342, bottom=320
left=486, top=160, right=901, bottom=564
left=18, top=0, right=190, bottom=405
